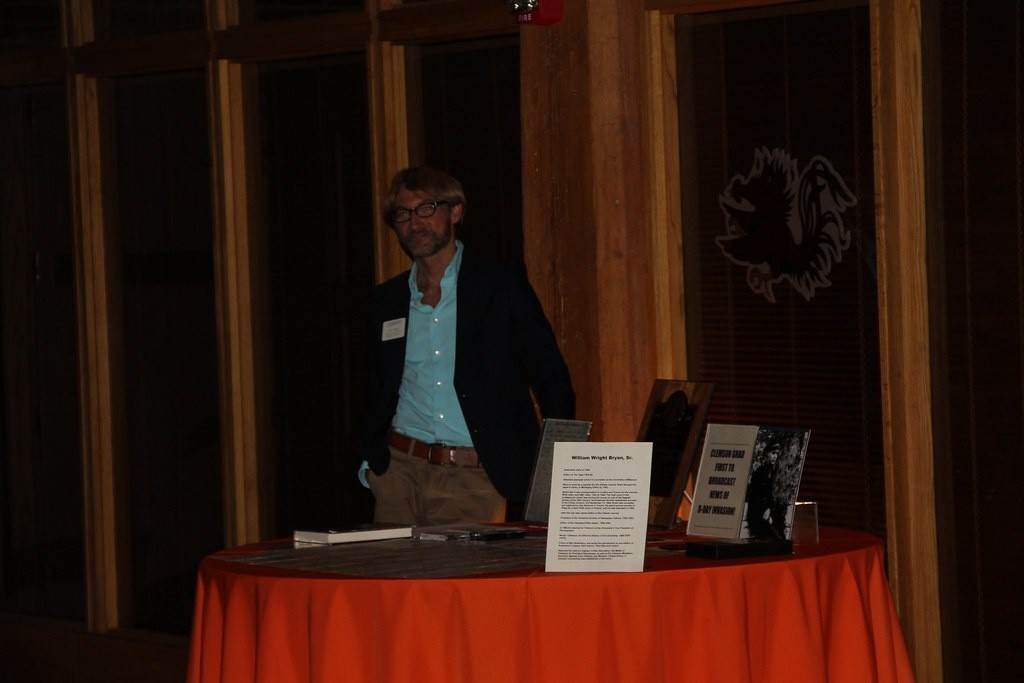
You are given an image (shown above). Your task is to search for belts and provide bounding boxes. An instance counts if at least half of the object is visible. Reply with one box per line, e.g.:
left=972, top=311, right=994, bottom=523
left=386, top=429, right=480, bottom=466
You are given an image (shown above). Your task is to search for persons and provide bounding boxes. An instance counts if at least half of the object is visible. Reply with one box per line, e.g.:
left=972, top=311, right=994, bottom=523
left=359, top=165, right=577, bottom=527
left=745, top=442, right=788, bottom=539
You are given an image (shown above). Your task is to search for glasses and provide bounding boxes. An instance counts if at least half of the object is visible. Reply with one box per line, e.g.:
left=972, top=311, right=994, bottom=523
left=389, top=200, right=455, bottom=223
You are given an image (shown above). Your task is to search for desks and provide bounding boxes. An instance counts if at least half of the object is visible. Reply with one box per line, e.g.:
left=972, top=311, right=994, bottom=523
left=186, top=519, right=916, bottom=682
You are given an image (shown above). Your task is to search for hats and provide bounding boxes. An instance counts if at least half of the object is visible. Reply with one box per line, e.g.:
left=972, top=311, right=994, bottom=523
left=763, top=442, right=783, bottom=454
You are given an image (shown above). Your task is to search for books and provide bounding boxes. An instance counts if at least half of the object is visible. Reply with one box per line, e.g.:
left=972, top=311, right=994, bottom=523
left=686, top=423, right=813, bottom=544
